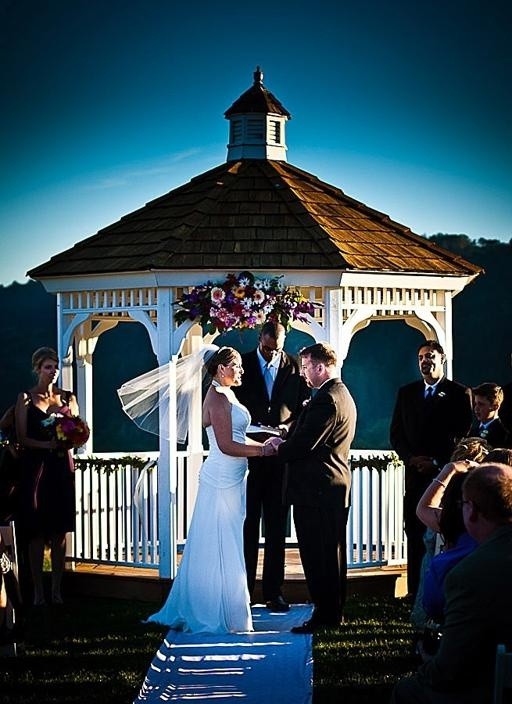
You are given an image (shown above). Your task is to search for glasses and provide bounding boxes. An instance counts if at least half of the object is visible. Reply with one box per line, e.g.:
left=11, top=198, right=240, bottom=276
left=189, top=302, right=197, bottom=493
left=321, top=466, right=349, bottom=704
left=456, top=498, right=468, bottom=506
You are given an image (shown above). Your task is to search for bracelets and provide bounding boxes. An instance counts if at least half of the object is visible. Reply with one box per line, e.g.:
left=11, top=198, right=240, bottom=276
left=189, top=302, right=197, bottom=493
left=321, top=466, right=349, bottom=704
left=259, top=446, right=266, bottom=458
left=432, top=476, right=449, bottom=489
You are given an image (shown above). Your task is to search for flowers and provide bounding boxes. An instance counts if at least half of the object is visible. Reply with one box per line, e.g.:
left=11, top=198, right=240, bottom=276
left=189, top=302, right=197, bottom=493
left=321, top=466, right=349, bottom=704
left=54, top=418, right=92, bottom=449
left=174, top=272, right=324, bottom=339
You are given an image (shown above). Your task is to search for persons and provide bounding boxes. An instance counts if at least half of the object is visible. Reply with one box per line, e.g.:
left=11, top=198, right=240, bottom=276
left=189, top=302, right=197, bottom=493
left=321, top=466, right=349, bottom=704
left=389, top=466, right=511, bottom=704
left=416, top=447, right=510, bottom=552
left=446, top=431, right=493, bottom=494
left=238, top=323, right=313, bottom=610
left=391, top=341, right=472, bottom=605
left=16, top=346, right=87, bottom=611
left=118, top=341, right=275, bottom=635
left=457, top=383, right=512, bottom=461
left=266, top=342, right=356, bottom=632
left=422, top=474, right=484, bottom=622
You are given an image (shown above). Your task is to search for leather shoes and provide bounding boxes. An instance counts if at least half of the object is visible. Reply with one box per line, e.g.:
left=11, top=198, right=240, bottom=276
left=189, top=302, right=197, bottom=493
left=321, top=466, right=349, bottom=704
left=266, top=593, right=291, bottom=612
left=289, top=617, right=339, bottom=634
left=32, top=597, right=51, bottom=609
left=51, top=593, right=65, bottom=610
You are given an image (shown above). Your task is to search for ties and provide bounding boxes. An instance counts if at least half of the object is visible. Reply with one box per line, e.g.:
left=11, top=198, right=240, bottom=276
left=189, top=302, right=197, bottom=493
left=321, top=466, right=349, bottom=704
left=264, top=364, right=275, bottom=398
left=424, top=385, right=433, bottom=406
